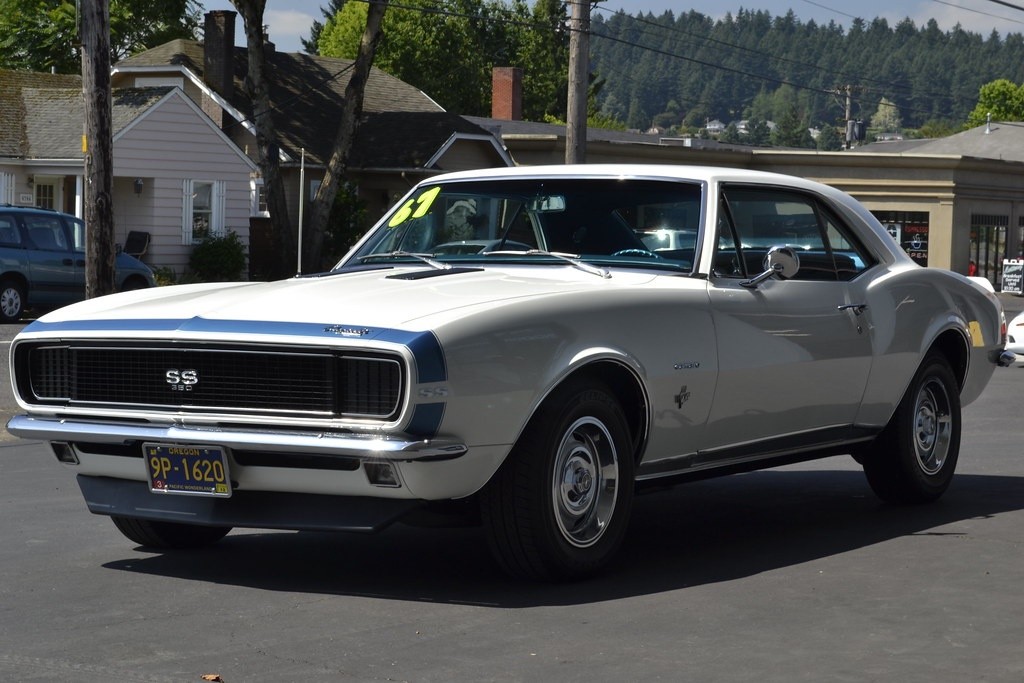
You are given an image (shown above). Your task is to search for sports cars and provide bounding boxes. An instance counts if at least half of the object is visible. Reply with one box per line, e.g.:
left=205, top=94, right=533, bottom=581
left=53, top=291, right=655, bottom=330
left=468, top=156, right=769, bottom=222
left=2, top=161, right=1016, bottom=584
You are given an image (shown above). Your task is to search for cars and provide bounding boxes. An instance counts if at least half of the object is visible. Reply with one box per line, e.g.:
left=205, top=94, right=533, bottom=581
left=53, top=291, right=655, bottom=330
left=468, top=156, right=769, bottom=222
left=0, top=203, right=158, bottom=323
left=1005, top=310, right=1024, bottom=367
